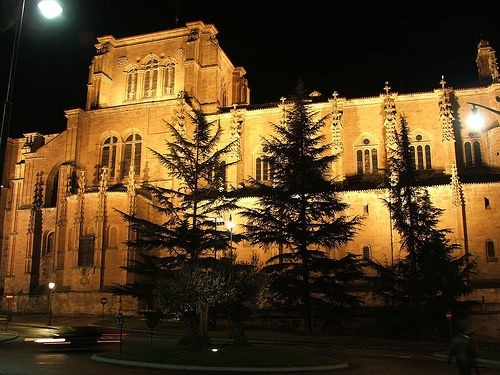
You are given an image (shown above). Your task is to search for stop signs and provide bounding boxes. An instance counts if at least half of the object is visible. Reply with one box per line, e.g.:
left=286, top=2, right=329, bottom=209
left=446, top=311, right=452, bottom=320
left=6, top=293, right=14, bottom=300
left=100, top=297, right=108, bottom=304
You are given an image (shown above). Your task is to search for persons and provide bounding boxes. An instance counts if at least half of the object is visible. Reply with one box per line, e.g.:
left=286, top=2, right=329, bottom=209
left=447, top=326, right=481, bottom=375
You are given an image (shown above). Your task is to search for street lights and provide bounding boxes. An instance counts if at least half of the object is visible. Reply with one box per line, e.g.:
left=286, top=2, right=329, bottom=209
left=2, top=1, right=64, bottom=191
left=47, top=282, right=55, bottom=326
